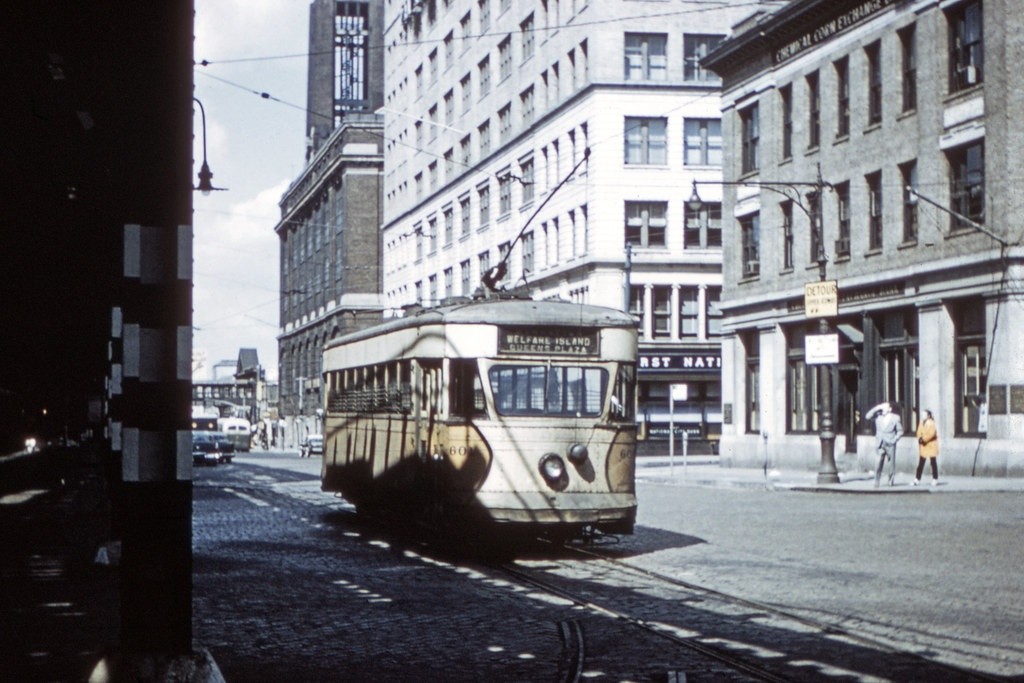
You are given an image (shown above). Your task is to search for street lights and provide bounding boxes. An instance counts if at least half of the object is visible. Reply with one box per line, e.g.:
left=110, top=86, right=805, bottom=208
left=685, top=181, right=841, bottom=485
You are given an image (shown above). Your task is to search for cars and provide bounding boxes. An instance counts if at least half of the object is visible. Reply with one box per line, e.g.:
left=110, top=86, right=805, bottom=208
left=193, top=434, right=235, bottom=465
left=302, top=436, right=323, bottom=458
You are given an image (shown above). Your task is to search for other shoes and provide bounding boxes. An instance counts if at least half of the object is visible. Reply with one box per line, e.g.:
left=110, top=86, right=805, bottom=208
left=874, top=482, right=881, bottom=487
left=910, top=478, right=921, bottom=487
left=888, top=479, right=894, bottom=487
left=930, top=479, right=939, bottom=489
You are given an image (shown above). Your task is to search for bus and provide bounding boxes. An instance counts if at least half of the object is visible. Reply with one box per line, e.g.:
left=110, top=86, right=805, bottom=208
left=320, top=296, right=639, bottom=536
left=215, top=418, right=251, bottom=451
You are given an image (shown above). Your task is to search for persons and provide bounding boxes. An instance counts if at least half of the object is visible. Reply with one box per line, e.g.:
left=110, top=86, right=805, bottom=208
left=909, top=410, right=940, bottom=487
left=865, top=401, right=904, bottom=487
